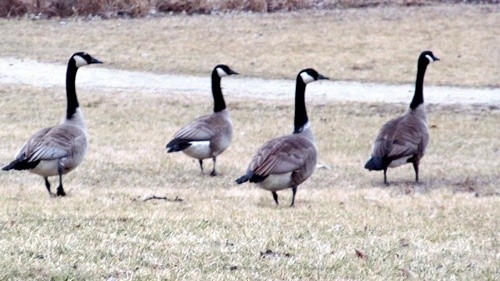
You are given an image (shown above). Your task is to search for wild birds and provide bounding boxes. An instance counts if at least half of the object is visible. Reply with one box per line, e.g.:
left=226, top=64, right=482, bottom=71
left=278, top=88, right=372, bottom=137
left=234, top=68, right=330, bottom=207
left=364, top=50, right=440, bottom=186
left=1, top=51, right=102, bottom=197
left=165, top=64, right=239, bottom=176
